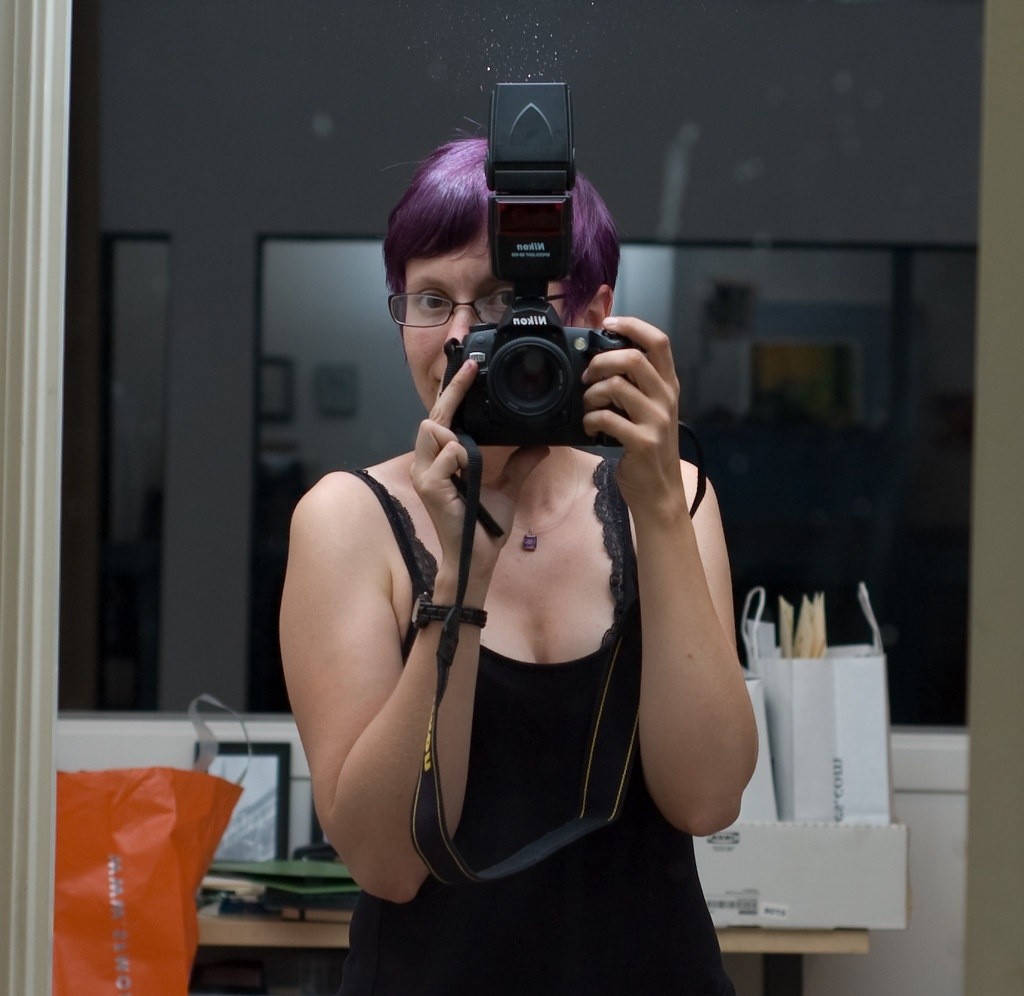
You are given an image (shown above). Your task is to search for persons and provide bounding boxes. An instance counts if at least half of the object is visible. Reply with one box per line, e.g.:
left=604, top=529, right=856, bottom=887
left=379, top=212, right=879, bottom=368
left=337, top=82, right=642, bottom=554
left=281, top=138, right=760, bottom=996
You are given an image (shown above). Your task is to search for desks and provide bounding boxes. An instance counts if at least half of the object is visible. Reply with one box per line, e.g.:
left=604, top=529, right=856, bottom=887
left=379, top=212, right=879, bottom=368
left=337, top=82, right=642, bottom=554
left=197, top=891, right=876, bottom=995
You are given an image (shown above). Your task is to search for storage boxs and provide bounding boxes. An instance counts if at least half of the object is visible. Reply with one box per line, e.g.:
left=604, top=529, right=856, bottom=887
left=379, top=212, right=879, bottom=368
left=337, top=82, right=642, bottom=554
left=688, top=819, right=909, bottom=934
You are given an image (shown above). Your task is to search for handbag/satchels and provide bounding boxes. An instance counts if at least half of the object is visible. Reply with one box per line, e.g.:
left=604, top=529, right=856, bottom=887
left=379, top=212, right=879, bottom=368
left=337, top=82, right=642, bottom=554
left=711, top=582, right=896, bottom=825
left=53, top=691, right=253, bottom=995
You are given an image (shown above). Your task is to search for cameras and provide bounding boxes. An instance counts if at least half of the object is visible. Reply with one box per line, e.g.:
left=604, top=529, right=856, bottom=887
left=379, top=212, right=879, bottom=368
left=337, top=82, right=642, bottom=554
left=440, top=84, right=647, bottom=447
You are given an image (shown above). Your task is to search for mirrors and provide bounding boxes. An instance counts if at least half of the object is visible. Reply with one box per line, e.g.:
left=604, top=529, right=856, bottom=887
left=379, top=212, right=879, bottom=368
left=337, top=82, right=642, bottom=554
left=49, top=0, right=1024, bottom=995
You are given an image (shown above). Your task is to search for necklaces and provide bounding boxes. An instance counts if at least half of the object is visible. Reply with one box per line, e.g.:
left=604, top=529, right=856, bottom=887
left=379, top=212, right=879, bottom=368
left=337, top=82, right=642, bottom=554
left=511, top=446, right=580, bottom=552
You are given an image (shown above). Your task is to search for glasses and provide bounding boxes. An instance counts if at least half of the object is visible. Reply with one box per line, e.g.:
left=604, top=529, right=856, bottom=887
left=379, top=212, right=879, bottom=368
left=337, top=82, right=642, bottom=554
left=387, top=288, right=570, bottom=328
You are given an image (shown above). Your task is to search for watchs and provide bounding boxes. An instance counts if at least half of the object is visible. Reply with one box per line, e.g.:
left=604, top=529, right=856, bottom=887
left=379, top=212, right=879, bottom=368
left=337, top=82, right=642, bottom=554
left=409, top=594, right=488, bottom=634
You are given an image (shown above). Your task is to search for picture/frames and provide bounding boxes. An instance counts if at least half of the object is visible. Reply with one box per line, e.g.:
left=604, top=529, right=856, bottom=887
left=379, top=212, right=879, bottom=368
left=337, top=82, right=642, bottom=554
left=193, top=742, right=290, bottom=866
left=260, top=353, right=295, bottom=427
left=741, top=334, right=866, bottom=426
left=312, top=361, right=360, bottom=415
